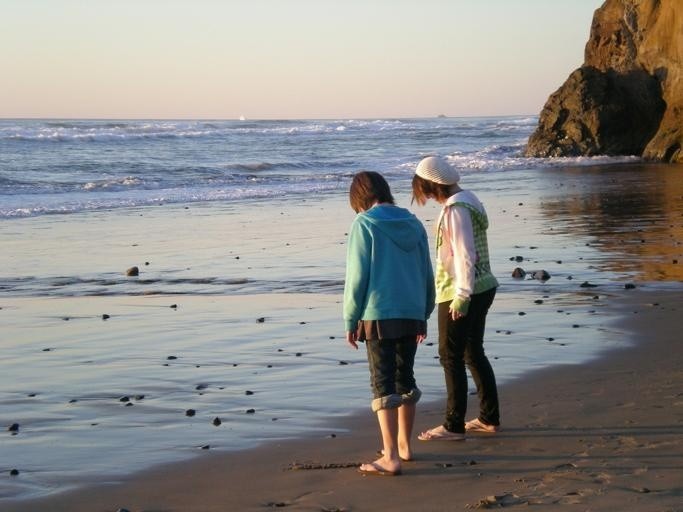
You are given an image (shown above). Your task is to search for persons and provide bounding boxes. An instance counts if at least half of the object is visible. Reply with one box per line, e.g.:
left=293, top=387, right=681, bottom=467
left=410, top=156, right=502, bottom=443
left=341, top=170, right=436, bottom=476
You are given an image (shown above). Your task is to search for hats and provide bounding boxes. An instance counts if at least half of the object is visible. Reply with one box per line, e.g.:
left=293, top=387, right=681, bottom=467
left=416, top=156, right=459, bottom=186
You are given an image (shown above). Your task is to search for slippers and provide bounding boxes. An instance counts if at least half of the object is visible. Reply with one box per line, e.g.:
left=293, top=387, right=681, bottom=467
left=417, top=425, right=465, bottom=442
left=463, top=418, right=497, bottom=433
left=373, top=446, right=413, bottom=461
left=356, top=461, right=399, bottom=476
